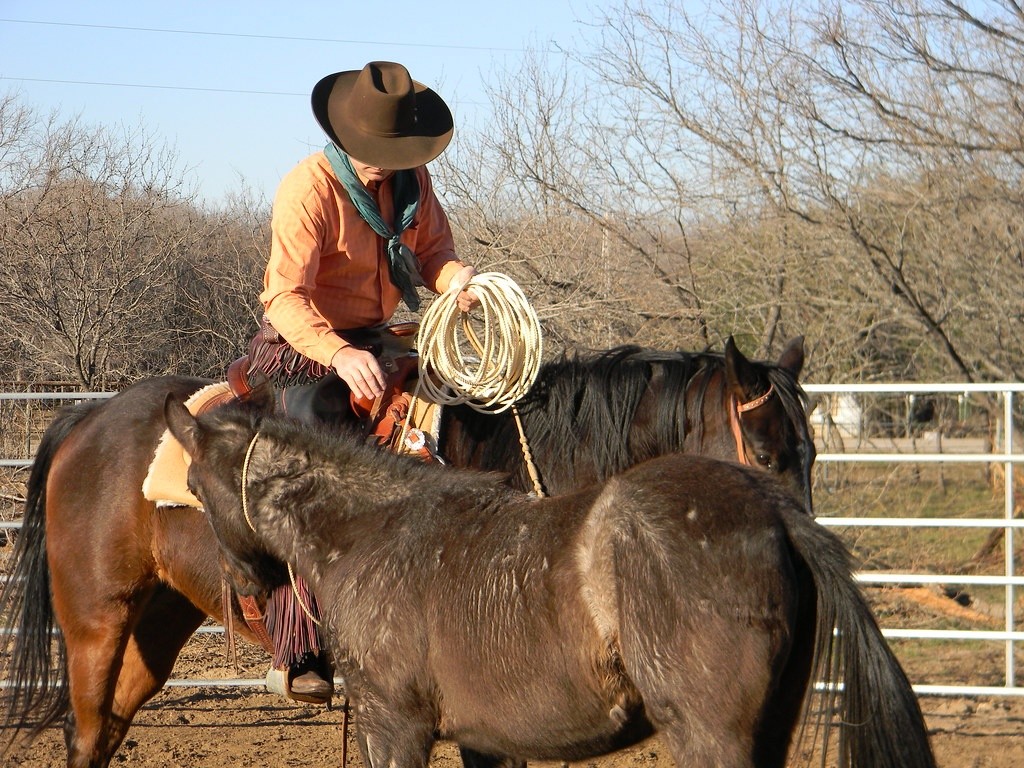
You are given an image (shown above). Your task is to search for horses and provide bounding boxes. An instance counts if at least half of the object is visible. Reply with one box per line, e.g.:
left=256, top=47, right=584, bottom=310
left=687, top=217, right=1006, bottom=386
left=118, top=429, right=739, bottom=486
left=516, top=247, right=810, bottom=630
left=167, top=370, right=940, bottom=768
left=2, top=332, right=816, bottom=768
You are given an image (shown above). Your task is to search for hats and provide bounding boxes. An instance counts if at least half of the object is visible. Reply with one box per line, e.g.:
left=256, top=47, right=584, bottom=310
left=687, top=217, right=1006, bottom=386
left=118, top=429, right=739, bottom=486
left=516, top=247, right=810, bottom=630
left=311, top=62, right=453, bottom=171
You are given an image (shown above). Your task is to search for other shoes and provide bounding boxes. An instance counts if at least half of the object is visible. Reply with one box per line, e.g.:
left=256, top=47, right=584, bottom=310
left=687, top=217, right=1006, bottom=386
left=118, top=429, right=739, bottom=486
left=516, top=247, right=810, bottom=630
left=289, top=653, right=331, bottom=698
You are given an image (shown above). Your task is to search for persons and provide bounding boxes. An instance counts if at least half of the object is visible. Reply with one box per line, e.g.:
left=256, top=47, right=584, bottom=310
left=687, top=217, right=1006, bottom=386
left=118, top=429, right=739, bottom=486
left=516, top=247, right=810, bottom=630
left=255, top=61, right=483, bottom=705
